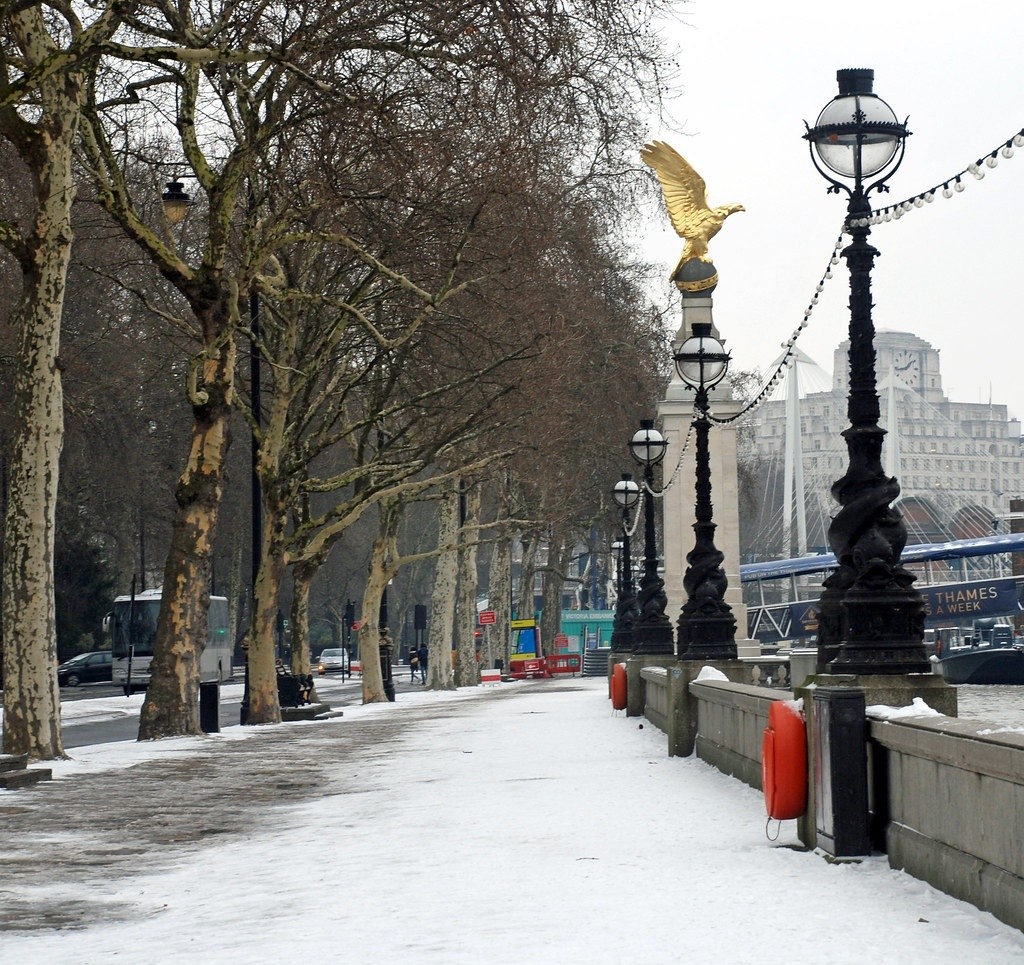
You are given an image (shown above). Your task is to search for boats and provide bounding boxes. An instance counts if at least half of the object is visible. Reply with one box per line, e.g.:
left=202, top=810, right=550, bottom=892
left=933, top=621, right=1024, bottom=686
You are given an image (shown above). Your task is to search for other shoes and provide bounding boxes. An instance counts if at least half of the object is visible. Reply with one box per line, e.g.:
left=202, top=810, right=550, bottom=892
left=421, top=682, right=425, bottom=684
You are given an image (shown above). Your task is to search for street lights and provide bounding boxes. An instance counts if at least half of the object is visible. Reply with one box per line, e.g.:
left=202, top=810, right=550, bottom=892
left=610, top=475, right=639, bottom=651
left=799, top=66, right=932, bottom=682
left=676, top=324, right=743, bottom=659
left=628, top=419, right=674, bottom=656
left=611, top=538, right=626, bottom=606
left=140, top=419, right=158, bottom=591
left=160, top=162, right=263, bottom=726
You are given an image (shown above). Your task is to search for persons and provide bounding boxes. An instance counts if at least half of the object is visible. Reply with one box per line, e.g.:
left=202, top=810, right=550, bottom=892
left=409, top=641, right=428, bottom=684
left=406, top=645, right=419, bottom=683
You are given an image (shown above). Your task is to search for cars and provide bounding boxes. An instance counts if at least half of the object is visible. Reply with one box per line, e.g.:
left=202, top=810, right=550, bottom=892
left=316, top=648, right=350, bottom=675
left=57, top=650, right=113, bottom=687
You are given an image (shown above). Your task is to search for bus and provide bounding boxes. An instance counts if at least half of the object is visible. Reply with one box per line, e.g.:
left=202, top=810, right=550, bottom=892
left=101, top=585, right=232, bottom=695
left=474, top=619, right=536, bottom=664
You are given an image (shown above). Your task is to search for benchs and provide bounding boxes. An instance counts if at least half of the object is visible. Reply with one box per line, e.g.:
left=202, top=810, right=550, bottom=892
left=276, top=660, right=314, bottom=708
